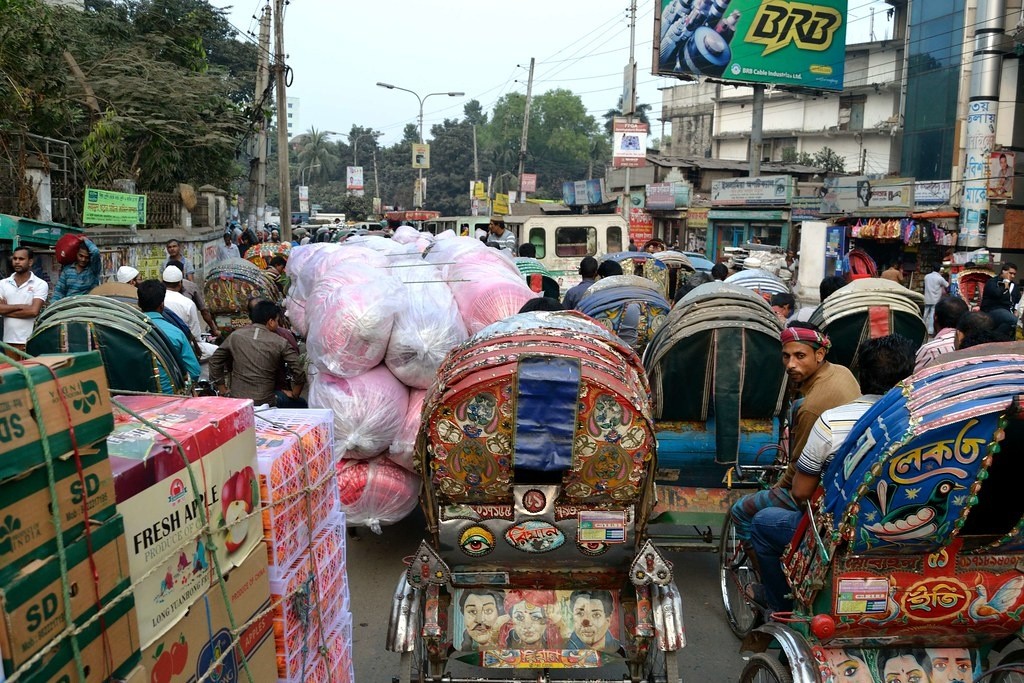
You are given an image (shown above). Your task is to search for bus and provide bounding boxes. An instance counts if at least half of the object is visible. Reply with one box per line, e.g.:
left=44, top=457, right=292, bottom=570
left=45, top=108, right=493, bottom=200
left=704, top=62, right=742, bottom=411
left=424, top=216, right=491, bottom=246
left=501, top=216, right=631, bottom=302
left=385, top=210, right=440, bottom=233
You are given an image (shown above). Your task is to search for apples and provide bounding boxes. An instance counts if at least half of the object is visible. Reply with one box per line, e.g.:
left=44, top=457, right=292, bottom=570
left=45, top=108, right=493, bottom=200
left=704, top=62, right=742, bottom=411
left=222, top=466, right=256, bottom=552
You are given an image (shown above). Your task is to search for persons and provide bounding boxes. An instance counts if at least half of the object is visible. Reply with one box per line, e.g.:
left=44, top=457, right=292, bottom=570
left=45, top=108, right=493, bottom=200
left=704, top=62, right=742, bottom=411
left=776, top=248, right=799, bottom=284
left=461, top=216, right=549, bottom=276
left=350, top=177, right=354, bottom=185
left=629, top=235, right=683, bottom=254
left=857, top=181, right=870, bottom=207
left=692, top=247, right=704, bottom=257
left=914, top=297, right=1017, bottom=374
left=771, top=276, right=848, bottom=324
left=671, top=258, right=761, bottom=307
left=923, top=263, right=951, bottom=335
left=998, top=155, right=1012, bottom=194
left=731, top=321, right=917, bottom=612
left=881, top=259, right=904, bottom=283
left=0, top=206, right=414, bottom=409
left=519, top=257, right=639, bottom=352
left=979, top=263, right=1021, bottom=313
left=558, top=278, right=567, bottom=293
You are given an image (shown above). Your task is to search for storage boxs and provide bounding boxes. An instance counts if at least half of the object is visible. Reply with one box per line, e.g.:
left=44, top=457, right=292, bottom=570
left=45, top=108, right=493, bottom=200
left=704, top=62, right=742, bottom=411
left=1, top=351, right=355, bottom=683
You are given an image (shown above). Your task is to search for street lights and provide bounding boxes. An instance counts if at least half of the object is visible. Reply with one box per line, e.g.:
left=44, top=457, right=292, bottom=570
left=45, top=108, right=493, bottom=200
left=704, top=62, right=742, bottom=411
left=376, top=82, right=465, bottom=232
left=325, top=130, right=385, bottom=168
left=302, top=165, right=321, bottom=186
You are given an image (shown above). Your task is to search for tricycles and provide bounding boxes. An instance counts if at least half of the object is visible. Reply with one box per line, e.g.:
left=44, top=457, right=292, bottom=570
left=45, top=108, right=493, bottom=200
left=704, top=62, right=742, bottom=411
left=25, top=243, right=933, bottom=559
left=717, top=344, right=1023, bottom=683
left=385, top=310, right=687, bottom=683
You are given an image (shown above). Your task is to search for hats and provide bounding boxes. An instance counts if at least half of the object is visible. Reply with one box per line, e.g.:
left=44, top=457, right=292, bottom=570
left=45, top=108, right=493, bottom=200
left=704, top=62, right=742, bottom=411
left=117, top=266, right=139, bottom=283
left=491, top=214, right=503, bottom=221
left=743, top=258, right=761, bottom=270
left=162, top=265, right=183, bottom=282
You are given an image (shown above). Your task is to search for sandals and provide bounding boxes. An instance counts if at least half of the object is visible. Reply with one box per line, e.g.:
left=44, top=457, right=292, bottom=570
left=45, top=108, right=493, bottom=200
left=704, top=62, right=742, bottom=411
left=745, top=582, right=767, bottom=605
